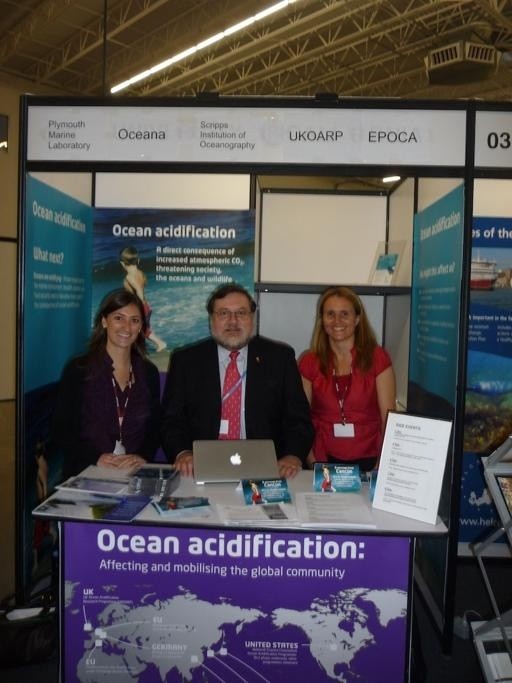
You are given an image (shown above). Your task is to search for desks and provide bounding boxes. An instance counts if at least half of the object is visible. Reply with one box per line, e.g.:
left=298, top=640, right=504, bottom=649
left=30, top=462, right=449, bottom=682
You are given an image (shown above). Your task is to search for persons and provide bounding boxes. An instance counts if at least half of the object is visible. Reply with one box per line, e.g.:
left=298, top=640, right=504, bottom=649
left=296, top=285, right=397, bottom=477
left=118, top=245, right=167, bottom=353
left=31, top=439, right=57, bottom=575
left=58, top=288, right=160, bottom=486
left=249, top=478, right=268, bottom=506
left=319, top=464, right=331, bottom=493
left=159, top=282, right=313, bottom=480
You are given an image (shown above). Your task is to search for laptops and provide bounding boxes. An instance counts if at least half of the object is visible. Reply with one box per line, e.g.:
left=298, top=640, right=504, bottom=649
left=191, top=438, right=281, bottom=486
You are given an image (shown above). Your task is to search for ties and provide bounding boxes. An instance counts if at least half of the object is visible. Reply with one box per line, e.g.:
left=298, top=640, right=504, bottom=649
left=219, top=351, right=242, bottom=440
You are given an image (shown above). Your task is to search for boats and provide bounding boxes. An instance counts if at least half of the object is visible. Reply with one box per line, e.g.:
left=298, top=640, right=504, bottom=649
left=470, top=249, right=503, bottom=289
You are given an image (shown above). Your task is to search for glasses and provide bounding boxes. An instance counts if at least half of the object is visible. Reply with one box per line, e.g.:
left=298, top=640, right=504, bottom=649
left=213, top=306, right=252, bottom=320
